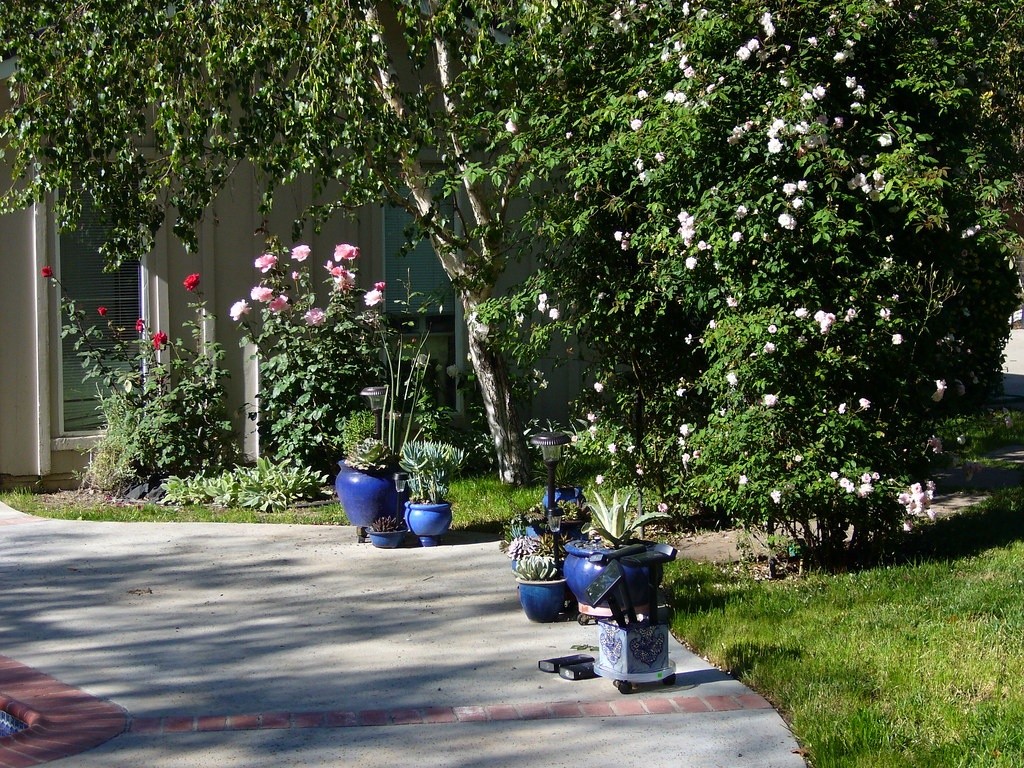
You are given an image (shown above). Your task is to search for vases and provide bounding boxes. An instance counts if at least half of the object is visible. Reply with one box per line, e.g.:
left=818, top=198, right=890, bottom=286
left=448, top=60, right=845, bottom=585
left=593, top=618, right=676, bottom=694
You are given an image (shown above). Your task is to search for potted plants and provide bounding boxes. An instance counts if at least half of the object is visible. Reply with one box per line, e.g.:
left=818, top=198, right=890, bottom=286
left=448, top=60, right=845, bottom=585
left=498, top=458, right=673, bottom=625
left=336, top=437, right=469, bottom=548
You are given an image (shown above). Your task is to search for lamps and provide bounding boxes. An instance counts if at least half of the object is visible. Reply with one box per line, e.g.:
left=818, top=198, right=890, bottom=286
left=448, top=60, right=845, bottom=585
left=360, top=386, right=389, bottom=411
left=531, top=433, right=572, bottom=464
